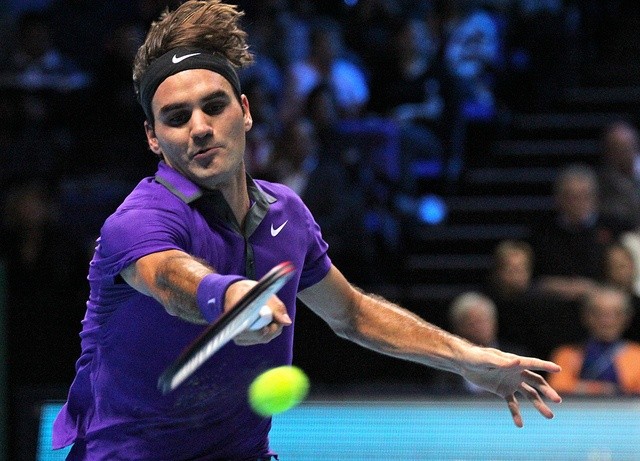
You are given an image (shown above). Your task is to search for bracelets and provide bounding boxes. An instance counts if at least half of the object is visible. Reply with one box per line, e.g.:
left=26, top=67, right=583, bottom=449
left=195, top=272, right=244, bottom=326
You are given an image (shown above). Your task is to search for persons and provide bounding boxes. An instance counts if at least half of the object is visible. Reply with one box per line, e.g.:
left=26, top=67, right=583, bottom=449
left=597, top=122, right=639, bottom=229
left=530, top=162, right=624, bottom=281
left=236, top=0, right=534, bottom=128
left=544, top=288, right=640, bottom=398
left=54, top=0, right=562, bottom=461
left=482, top=239, right=558, bottom=360
left=2, top=2, right=160, bottom=404
left=426, top=293, right=534, bottom=401
left=535, top=239, right=639, bottom=310
left=243, top=128, right=500, bottom=275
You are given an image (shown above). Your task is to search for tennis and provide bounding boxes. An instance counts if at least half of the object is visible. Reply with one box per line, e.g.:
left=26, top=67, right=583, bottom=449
left=249, top=366, right=309, bottom=418
